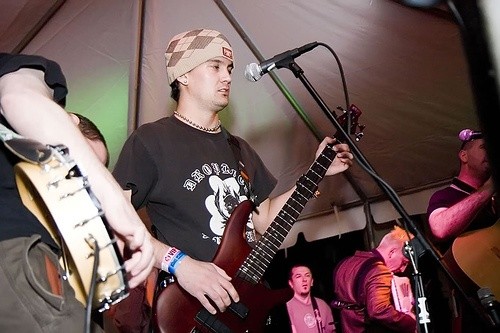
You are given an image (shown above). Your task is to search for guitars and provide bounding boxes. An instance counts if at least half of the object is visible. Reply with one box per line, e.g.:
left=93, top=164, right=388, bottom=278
left=443, top=217, right=500, bottom=312
left=153, top=103, right=362, bottom=333
left=13, top=143, right=131, bottom=315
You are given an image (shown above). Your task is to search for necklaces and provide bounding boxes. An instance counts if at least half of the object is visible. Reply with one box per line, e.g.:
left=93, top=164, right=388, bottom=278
left=173, top=110, right=222, bottom=132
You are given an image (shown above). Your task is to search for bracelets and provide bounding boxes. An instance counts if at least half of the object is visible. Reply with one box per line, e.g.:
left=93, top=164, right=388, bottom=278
left=168, top=251, right=187, bottom=274
left=161, top=247, right=180, bottom=273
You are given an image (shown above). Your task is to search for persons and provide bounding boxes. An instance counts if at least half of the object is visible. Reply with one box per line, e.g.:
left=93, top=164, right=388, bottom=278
left=335, top=224, right=416, bottom=333
left=427, top=129, right=500, bottom=333
left=112, top=27, right=354, bottom=333
left=286, top=264, right=336, bottom=333
left=0, top=53, right=157, bottom=333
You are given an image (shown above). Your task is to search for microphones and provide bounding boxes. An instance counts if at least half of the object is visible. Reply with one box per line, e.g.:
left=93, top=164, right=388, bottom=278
left=477, top=287, right=500, bottom=328
left=458, top=129, right=485, bottom=141
left=245, top=40, right=317, bottom=82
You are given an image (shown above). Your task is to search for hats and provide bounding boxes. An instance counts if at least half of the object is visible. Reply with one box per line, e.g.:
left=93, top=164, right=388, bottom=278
left=165, top=28, right=234, bottom=86
